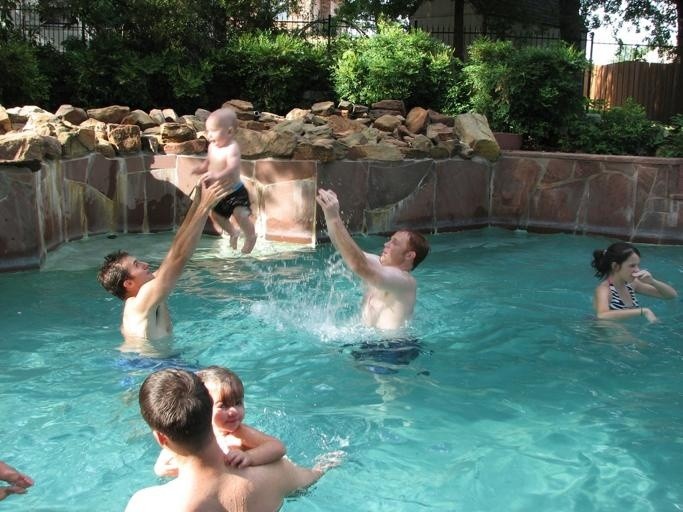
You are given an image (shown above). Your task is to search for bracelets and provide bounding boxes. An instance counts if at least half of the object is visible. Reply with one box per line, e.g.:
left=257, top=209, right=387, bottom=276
left=641, top=307, right=644, bottom=318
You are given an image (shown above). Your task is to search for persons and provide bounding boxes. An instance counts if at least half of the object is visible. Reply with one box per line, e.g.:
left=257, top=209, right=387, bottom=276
left=0, top=460, right=34, bottom=500
left=316, top=186, right=432, bottom=402
left=126, top=368, right=340, bottom=511
left=188, top=107, right=257, bottom=258
left=153, top=364, right=287, bottom=478
left=592, top=243, right=680, bottom=326
left=95, top=182, right=228, bottom=402
